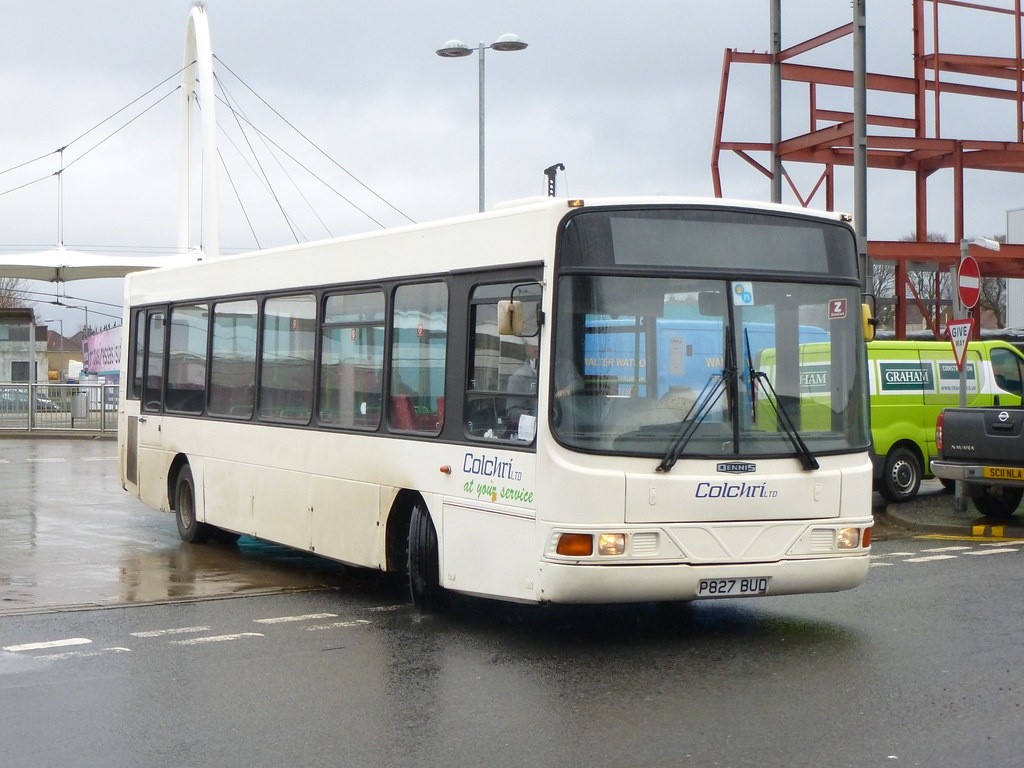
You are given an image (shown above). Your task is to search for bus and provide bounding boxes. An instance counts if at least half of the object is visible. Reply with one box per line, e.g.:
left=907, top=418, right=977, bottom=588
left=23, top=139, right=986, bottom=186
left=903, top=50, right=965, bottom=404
left=117, top=196, right=880, bottom=607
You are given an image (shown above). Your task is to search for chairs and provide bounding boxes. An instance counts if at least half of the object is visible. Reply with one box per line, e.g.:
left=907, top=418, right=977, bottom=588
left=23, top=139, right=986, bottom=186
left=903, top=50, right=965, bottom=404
left=164, top=351, right=444, bottom=434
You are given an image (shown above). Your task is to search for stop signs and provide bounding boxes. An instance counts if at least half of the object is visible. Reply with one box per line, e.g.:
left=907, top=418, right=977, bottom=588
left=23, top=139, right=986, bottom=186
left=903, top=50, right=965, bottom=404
left=958, top=256, right=981, bottom=308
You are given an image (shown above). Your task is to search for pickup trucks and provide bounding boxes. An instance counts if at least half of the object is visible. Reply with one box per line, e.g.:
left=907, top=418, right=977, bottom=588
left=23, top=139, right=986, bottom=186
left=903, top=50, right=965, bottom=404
left=931, top=392, right=1024, bottom=515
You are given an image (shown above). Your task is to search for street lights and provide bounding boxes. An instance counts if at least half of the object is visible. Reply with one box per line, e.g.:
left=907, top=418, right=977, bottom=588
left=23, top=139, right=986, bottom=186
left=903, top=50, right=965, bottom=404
left=435, top=32, right=529, bottom=213
left=65, top=306, right=88, bottom=338
left=44, top=319, right=63, bottom=384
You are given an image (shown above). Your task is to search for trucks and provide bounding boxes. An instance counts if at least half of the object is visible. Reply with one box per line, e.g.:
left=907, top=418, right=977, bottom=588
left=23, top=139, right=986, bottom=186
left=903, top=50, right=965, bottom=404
left=584, top=320, right=830, bottom=422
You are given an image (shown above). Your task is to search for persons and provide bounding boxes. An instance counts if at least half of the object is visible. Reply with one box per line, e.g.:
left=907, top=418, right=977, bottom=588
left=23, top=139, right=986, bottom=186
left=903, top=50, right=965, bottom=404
left=505, top=316, right=585, bottom=429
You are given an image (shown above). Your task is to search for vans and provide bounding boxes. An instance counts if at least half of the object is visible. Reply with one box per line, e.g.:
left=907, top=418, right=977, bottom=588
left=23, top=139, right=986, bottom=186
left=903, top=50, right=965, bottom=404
left=757, top=340, right=1024, bottom=503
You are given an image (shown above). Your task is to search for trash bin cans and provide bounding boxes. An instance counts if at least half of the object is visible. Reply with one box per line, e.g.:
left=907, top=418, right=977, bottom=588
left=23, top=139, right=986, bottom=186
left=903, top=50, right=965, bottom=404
left=71, top=391, right=90, bottom=419
